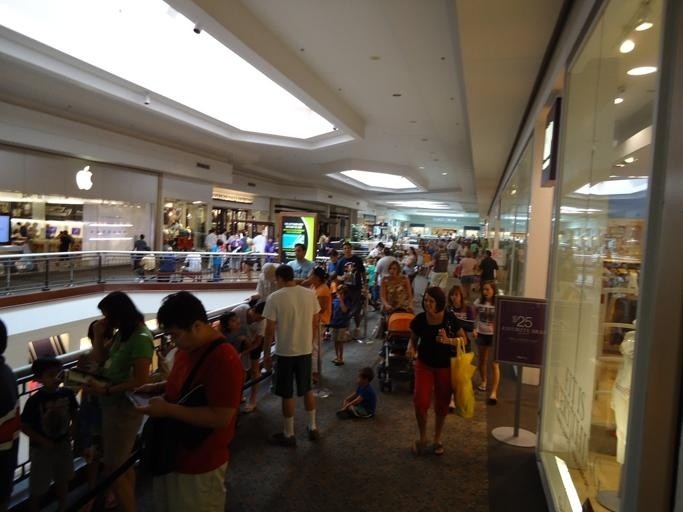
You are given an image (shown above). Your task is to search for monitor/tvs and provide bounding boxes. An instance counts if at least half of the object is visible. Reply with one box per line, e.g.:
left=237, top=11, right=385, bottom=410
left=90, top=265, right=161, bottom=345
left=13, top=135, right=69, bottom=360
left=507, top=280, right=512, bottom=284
left=0, top=213, right=11, bottom=245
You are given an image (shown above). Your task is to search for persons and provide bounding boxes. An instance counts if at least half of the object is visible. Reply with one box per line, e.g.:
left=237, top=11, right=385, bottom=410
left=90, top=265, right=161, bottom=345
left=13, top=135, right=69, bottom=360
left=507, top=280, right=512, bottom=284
left=610, top=321, right=638, bottom=465
left=1, top=290, right=262, bottom=511
left=263, top=218, right=509, bottom=457
left=131, top=220, right=260, bottom=284
left=10, top=220, right=74, bottom=273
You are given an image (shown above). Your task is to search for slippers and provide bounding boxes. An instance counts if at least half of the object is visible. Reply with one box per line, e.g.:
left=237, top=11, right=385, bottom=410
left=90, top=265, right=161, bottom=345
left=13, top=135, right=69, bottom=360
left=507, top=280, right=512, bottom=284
left=434, top=441, right=444, bottom=455
left=411, top=439, right=426, bottom=455
left=478, top=384, right=487, bottom=391
left=488, top=394, right=497, bottom=403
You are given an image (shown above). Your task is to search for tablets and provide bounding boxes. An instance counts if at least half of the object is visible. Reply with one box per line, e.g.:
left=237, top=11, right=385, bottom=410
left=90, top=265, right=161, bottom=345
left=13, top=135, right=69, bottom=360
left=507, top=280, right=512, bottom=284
left=122, top=389, right=150, bottom=407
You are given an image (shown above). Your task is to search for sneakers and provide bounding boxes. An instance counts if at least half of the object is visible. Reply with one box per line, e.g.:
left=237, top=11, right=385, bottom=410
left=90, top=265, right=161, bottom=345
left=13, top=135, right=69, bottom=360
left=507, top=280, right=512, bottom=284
left=331, top=358, right=344, bottom=366
left=266, top=434, right=295, bottom=446
left=336, top=410, right=352, bottom=420
left=307, top=423, right=320, bottom=441
left=244, top=403, right=256, bottom=414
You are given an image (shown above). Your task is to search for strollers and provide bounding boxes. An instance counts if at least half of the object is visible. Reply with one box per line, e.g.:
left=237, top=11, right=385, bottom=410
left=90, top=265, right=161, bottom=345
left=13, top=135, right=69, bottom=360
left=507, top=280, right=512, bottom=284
left=378, top=307, right=416, bottom=395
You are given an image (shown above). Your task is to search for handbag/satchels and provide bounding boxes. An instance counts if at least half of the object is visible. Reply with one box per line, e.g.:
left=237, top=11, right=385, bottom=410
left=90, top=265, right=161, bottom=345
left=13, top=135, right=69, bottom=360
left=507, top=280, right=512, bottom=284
left=372, top=319, right=387, bottom=339
left=451, top=352, right=476, bottom=417
left=141, top=416, right=168, bottom=474
left=130, top=350, right=168, bottom=391
